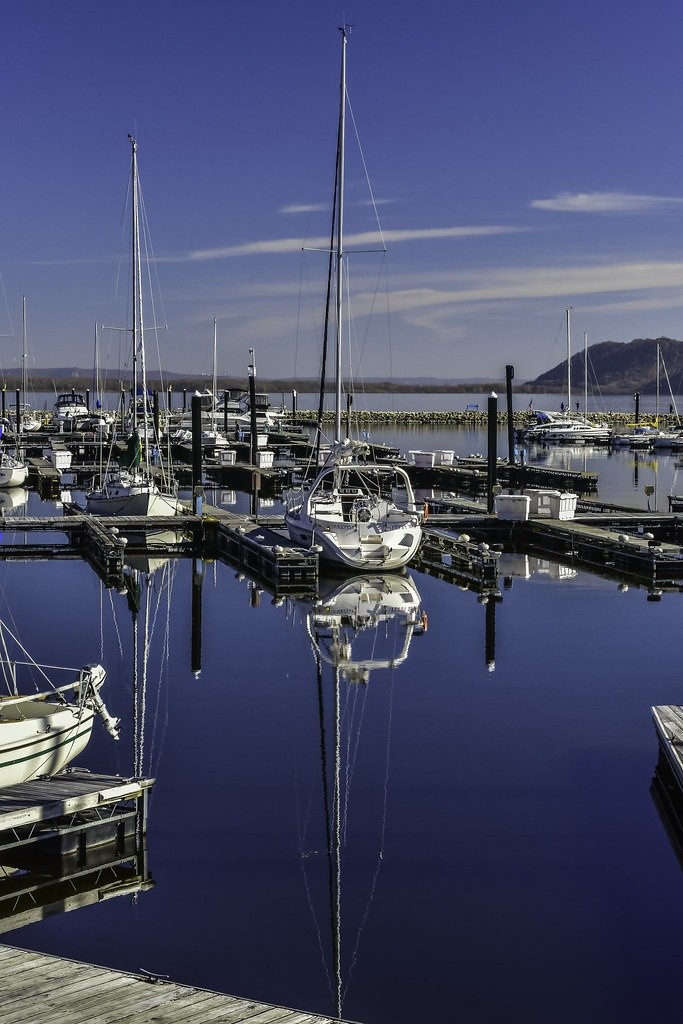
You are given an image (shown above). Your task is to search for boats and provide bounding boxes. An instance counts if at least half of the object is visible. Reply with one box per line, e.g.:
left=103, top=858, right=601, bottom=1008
left=192, top=315, right=286, bottom=447
left=0, top=620, right=125, bottom=793
left=0, top=426, right=29, bottom=486
left=0, top=487, right=31, bottom=525
left=51, top=382, right=94, bottom=433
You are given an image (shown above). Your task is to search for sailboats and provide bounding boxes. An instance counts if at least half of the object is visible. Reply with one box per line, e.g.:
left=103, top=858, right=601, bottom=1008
left=516, top=304, right=683, bottom=451
left=296, top=567, right=432, bottom=1017
left=275, top=19, right=431, bottom=575
left=7, top=293, right=44, bottom=432
left=97, top=528, right=181, bottom=782
left=77, top=124, right=187, bottom=517
